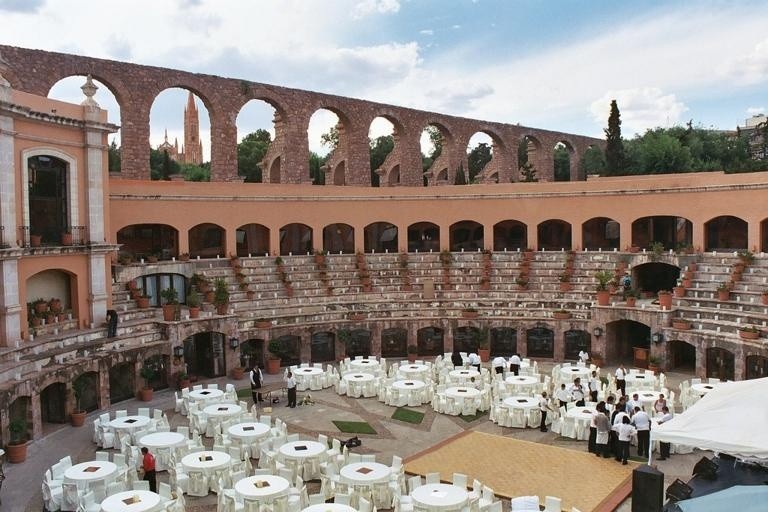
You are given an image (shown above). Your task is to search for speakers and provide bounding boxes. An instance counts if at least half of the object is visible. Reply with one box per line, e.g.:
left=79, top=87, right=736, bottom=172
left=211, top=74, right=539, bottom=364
left=666, top=478, right=694, bottom=500
left=632, top=464, right=664, bottom=512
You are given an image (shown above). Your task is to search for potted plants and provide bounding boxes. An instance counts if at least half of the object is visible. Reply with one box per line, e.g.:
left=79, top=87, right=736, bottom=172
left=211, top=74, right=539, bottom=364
left=6, top=418, right=29, bottom=463
left=69, top=376, right=92, bottom=426
left=138, top=357, right=156, bottom=400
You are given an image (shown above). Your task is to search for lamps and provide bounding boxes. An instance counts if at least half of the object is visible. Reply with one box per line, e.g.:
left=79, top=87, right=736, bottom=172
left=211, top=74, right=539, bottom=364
left=174, top=345, right=184, bottom=361
left=665, top=478, right=693, bottom=503
left=230, top=336, right=239, bottom=351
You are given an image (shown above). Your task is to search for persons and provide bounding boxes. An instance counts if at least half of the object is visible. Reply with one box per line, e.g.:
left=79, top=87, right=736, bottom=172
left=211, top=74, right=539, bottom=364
left=141, top=446, right=156, bottom=492
left=509, top=355, right=521, bottom=375
left=467, top=352, right=481, bottom=375
left=539, top=363, right=672, bottom=465
left=250, top=364, right=264, bottom=403
left=285, top=371, right=296, bottom=408
left=579, top=348, right=589, bottom=367
left=494, top=357, right=507, bottom=375
left=622, top=273, right=632, bottom=291
left=106, top=310, right=118, bottom=336
left=450, top=350, right=463, bottom=370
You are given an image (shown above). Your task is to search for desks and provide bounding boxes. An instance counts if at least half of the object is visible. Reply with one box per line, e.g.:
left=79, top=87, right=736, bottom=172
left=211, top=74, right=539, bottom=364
left=251, top=383, right=287, bottom=409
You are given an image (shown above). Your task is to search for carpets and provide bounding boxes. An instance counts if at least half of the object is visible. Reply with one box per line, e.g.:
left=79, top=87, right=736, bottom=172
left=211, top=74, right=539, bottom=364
left=393, top=430, right=657, bottom=512
